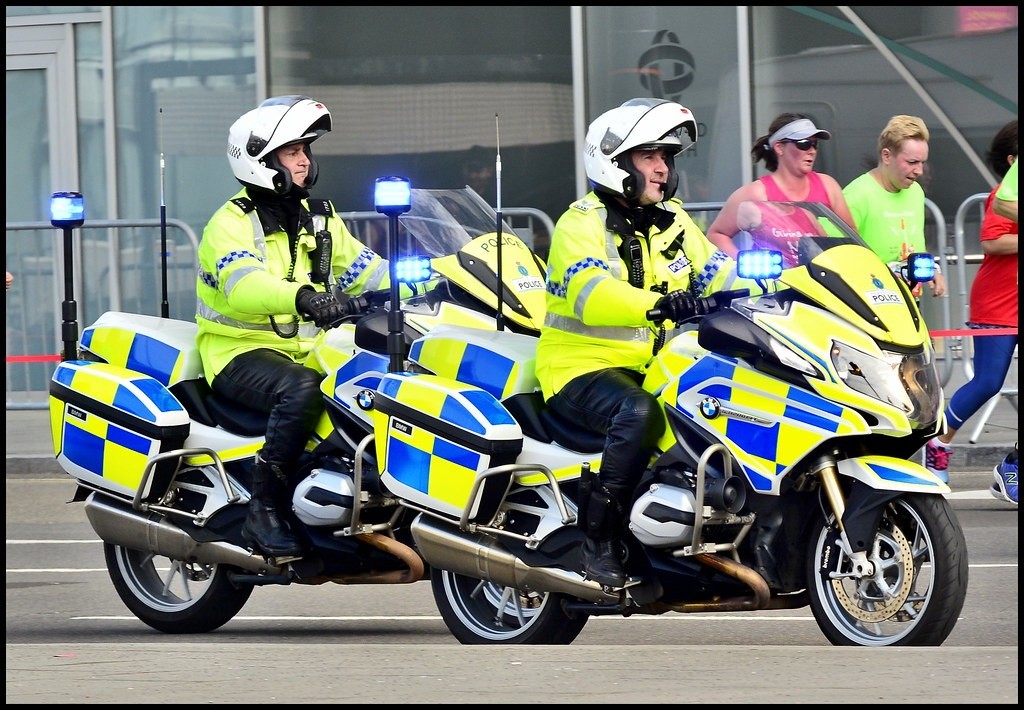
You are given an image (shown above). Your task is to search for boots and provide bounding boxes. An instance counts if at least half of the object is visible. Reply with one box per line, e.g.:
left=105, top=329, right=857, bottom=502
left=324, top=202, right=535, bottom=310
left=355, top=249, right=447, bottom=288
left=240, top=462, right=302, bottom=556
left=578, top=481, right=632, bottom=588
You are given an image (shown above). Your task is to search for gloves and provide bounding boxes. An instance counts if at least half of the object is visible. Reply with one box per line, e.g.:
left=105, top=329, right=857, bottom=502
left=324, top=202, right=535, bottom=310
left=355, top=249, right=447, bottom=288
left=297, top=289, right=344, bottom=328
left=655, top=289, right=699, bottom=324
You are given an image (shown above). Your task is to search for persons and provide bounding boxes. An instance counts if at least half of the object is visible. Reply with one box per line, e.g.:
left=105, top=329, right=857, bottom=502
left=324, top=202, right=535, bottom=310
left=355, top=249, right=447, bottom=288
left=842, top=115, right=944, bottom=311
left=706, top=112, right=859, bottom=270
left=922, top=121, right=1018, bottom=506
left=194, top=95, right=439, bottom=556
left=534, top=98, right=790, bottom=588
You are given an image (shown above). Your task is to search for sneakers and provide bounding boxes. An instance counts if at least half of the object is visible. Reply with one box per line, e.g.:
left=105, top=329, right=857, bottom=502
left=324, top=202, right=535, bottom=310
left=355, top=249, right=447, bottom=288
left=920, top=439, right=953, bottom=485
left=989, top=452, right=1018, bottom=506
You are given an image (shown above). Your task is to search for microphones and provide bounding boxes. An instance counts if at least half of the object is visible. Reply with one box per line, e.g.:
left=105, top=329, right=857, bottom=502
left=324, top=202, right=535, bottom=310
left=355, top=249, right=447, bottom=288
left=304, top=176, right=313, bottom=186
left=659, top=183, right=669, bottom=192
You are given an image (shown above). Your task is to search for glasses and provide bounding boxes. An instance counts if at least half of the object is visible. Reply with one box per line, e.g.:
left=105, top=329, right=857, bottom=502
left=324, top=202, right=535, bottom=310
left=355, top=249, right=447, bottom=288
left=781, top=138, right=818, bottom=150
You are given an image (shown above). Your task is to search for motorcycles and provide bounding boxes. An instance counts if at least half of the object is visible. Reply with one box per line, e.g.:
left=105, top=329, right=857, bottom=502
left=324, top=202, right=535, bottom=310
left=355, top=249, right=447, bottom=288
left=357, top=178, right=970, bottom=646
left=46, top=185, right=545, bottom=633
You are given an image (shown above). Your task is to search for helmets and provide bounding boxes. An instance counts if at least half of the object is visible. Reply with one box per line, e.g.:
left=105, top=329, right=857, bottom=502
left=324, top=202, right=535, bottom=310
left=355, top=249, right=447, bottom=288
left=226, top=97, right=333, bottom=195
left=581, top=101, right=699, bottom=202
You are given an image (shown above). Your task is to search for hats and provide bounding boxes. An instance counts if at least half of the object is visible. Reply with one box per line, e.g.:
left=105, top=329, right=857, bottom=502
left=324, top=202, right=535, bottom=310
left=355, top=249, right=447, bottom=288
left=763, top=119, right=831, bottom=151
